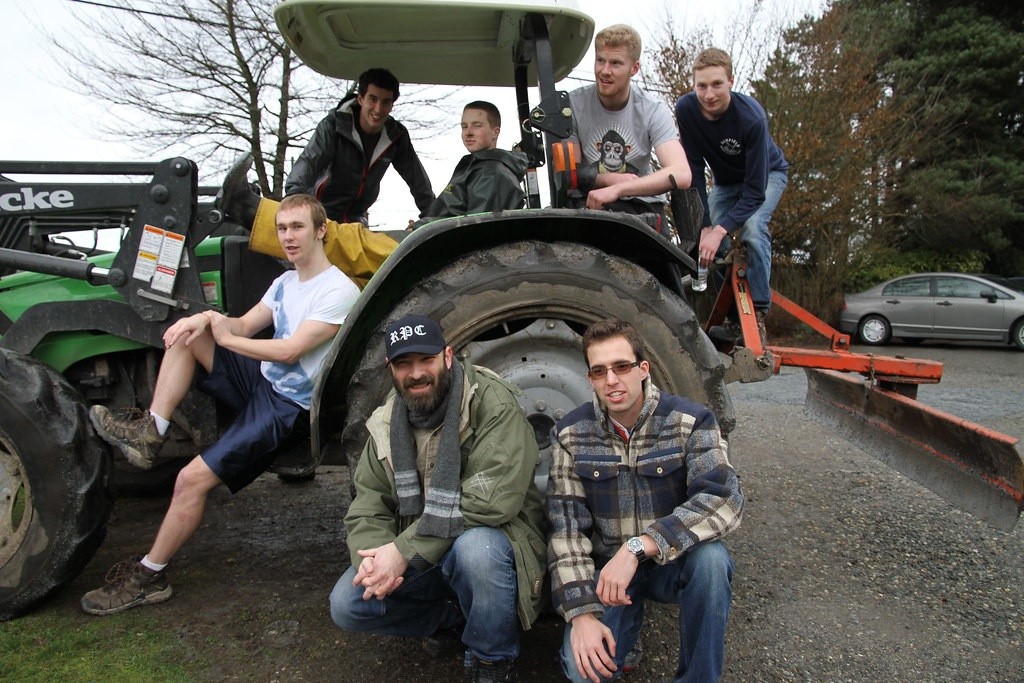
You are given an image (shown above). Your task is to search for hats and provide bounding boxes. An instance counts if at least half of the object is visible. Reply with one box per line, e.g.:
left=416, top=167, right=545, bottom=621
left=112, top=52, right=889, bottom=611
left=384, top=314, right=446, bottom=367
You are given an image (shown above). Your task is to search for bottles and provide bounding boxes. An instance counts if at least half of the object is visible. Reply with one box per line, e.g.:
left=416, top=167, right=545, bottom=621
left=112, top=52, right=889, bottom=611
left=692, top=255, right=708, bottom=291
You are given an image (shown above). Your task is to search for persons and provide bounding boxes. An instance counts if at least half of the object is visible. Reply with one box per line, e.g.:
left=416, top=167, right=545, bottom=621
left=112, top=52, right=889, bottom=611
left=81, top=194, right=362, bottom=615
left=285, top=68, right=436, bottom=230
left=329, top=316, right=552, bottom=683
left=676, top=48, right=788, bottom=349
left=563, top=24, right=693, bottom=311
left=215, top=101, right=529, bottom=290
left=543, top=319, right=745, bottom=683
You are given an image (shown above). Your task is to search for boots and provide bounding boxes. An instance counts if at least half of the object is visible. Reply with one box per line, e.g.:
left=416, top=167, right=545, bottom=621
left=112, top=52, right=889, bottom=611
left=213, top=151, right=261, bottom=231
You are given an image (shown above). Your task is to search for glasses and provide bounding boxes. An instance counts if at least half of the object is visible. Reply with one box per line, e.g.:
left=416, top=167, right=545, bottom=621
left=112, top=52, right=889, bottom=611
left=588, top=361, right=641, bottom=380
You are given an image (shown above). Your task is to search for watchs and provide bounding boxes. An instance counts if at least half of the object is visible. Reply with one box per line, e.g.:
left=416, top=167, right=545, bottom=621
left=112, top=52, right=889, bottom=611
left=627, top=536, right=646, bottom=563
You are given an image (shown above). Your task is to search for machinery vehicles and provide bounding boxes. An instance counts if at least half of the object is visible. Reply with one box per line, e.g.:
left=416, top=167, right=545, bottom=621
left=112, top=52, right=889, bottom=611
left=1, top=1, right=1023, bottom=627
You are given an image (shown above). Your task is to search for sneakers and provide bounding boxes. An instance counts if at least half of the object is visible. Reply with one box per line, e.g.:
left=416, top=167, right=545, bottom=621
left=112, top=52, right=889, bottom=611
left=420, top=612, right=523, bottom=682
left=707, top=311, right=767, bottom=348
left=80, top=550, right=175, bottom=616
left=88, top=403, right=174, bottom=472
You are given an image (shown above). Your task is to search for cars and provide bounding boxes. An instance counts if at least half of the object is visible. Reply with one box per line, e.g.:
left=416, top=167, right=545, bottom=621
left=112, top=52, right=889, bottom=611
left=837, top=271, right=1023, bottom=355
